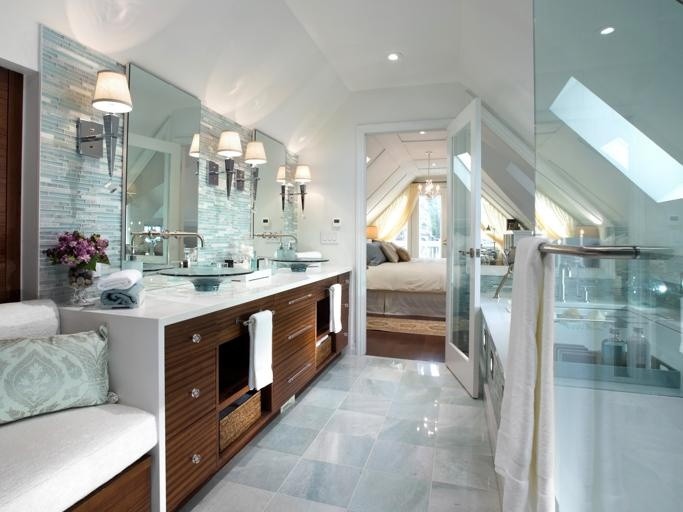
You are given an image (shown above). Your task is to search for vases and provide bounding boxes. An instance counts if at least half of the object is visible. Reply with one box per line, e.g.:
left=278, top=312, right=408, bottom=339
left=67, top=266, right=94, bottom=308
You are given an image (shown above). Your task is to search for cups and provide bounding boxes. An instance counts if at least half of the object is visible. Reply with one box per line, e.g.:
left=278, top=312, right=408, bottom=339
left=224, top=259, right=233, bottom=268
left=182, top=260, right=187, bottom=268
left=221, top=263, right=227, bottom=269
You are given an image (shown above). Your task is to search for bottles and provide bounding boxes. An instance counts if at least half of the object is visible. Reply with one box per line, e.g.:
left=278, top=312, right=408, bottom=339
left=627, top=327, right=651, bottom=370
left=184, top=251, right=190, bottom=268
left=601, top=325, right=626, bottom=366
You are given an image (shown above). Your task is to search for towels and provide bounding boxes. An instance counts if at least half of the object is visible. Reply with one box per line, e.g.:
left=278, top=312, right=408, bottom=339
left=246, top=309, right=274, bottom=391
left=492, top=235, right=559, bottom=512
left=98, top=270, right=142, bottom=291
left=328, top=283, right=343, bottom=334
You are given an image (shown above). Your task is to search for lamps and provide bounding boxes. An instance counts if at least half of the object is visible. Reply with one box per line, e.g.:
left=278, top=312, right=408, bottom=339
left=75, top=70, right=131, bottom=176
left=233, top=141, right=267, bottom=208
left=292, top=163, right=312, bottom=210
left=205, top=131, right=242, bottom=201
left=366, top=226, right=377, bottom=242
left=275, top=166, right=287, bottom=209
left=417, top=151, right=440, bottom=200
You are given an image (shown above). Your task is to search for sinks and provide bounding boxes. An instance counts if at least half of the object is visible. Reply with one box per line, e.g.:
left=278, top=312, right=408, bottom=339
left=271, top=255, right=329, bottom=273
left=158, top=262, right=255, bottom=291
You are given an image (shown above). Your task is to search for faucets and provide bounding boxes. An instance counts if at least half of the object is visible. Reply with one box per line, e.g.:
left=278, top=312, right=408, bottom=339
left=128, top=231, right=147, bottom=255
left=270, top=233, right=298, bottom=245
left=148, top=230, right=205, bottom=249
left=254, top=232, right=270, bottom=240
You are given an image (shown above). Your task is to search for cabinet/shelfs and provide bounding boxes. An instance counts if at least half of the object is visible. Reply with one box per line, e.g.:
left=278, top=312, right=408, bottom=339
left=164, top=314, right=219, bottom=512
left=269, top=283, right=316, bottom=412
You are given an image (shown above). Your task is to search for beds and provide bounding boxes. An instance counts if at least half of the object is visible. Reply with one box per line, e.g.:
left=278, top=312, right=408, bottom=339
left=366, top=242, right=447, bottom=338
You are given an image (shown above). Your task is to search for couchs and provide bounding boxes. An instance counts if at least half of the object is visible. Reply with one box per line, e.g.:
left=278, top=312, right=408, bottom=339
left=0, top=298, right=157, bottom=512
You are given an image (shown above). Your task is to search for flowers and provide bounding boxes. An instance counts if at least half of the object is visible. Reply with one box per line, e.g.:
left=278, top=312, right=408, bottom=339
left=42, top=225, right=110, bottom=286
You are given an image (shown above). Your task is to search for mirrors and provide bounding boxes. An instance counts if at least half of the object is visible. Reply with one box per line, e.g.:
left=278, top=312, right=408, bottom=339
left=118, top=63, right=201, bottom=273
left=249, top=128, right=293, bottom=251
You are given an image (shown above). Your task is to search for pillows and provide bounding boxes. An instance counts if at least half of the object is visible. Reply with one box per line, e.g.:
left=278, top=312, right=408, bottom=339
left=0, top=322, right=120, bottom=425
left=294, top=250, right=322, bottom=260
left=380, top=242, right=400, bottom=263
left=396, top=246, right=410, bottom=261
left=100, top=283, right=145, bottom=308
left=366, top=242, right=386, bottom=267
